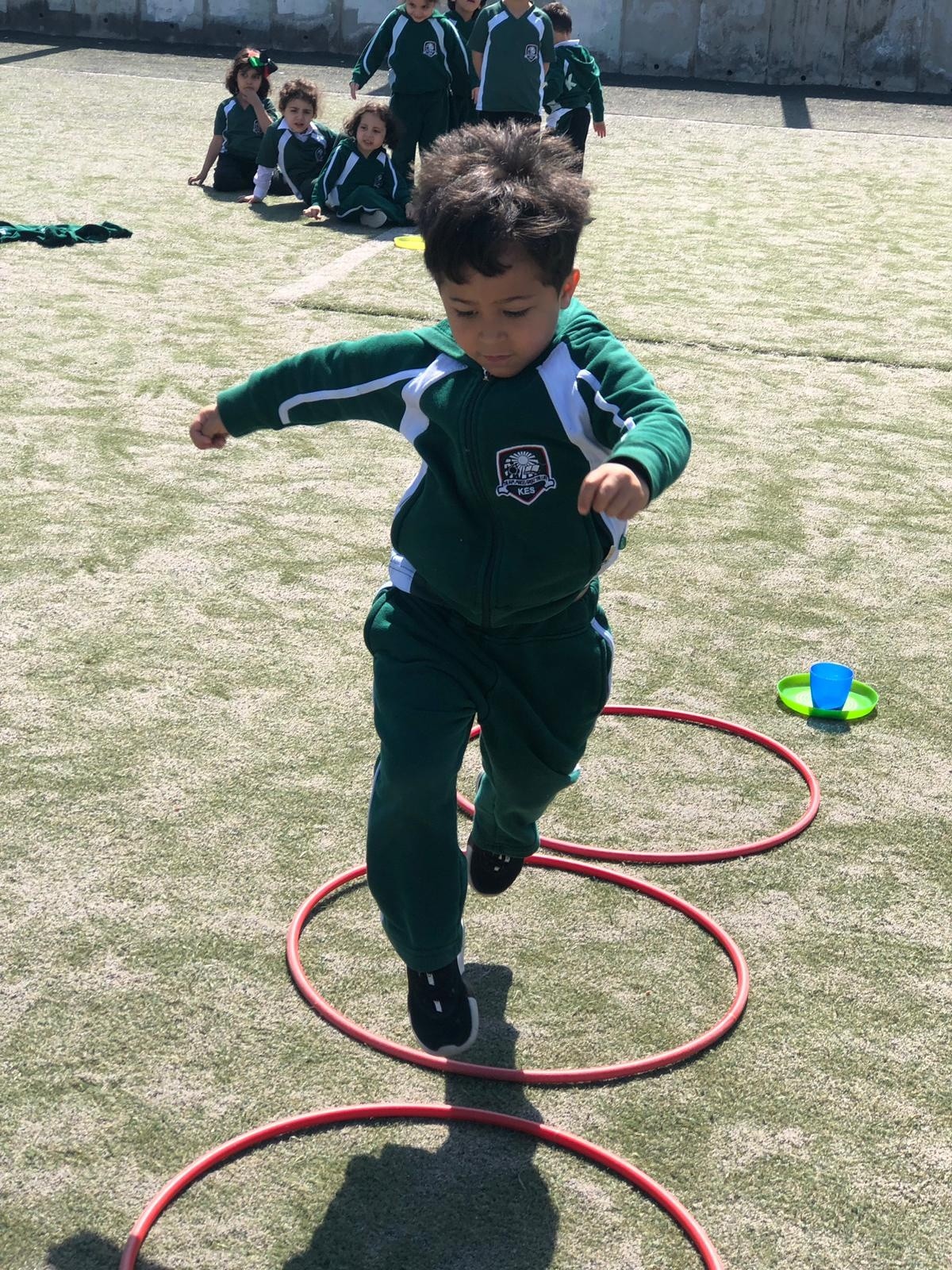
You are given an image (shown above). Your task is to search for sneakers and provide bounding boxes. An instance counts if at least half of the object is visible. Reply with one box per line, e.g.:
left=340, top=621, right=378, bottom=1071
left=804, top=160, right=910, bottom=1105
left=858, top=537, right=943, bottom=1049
left=467, top=771, right=524, bottom=896
left=406, top=920, right=480, bottom=1059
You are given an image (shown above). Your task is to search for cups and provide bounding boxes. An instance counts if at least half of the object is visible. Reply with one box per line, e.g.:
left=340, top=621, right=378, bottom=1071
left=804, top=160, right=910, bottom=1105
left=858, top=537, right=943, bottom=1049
left=810, top=662, right=854, bottom=711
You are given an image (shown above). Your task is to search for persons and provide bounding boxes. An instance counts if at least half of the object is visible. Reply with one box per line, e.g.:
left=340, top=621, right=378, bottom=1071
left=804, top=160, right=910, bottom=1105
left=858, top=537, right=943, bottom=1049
left=189, top=117, right=692, bottom=1058
left=304, top=100, right=418, bottom=228
left=188, top=48, right=294, bottom=196
left=237, top=77, right=339, bottom=213
left=350, top=0, right=604, bottom=229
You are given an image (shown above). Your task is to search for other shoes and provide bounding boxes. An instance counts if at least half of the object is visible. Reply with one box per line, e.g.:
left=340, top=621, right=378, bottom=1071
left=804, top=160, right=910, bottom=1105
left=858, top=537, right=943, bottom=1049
left=359, top=211, right=388, bottom=229
